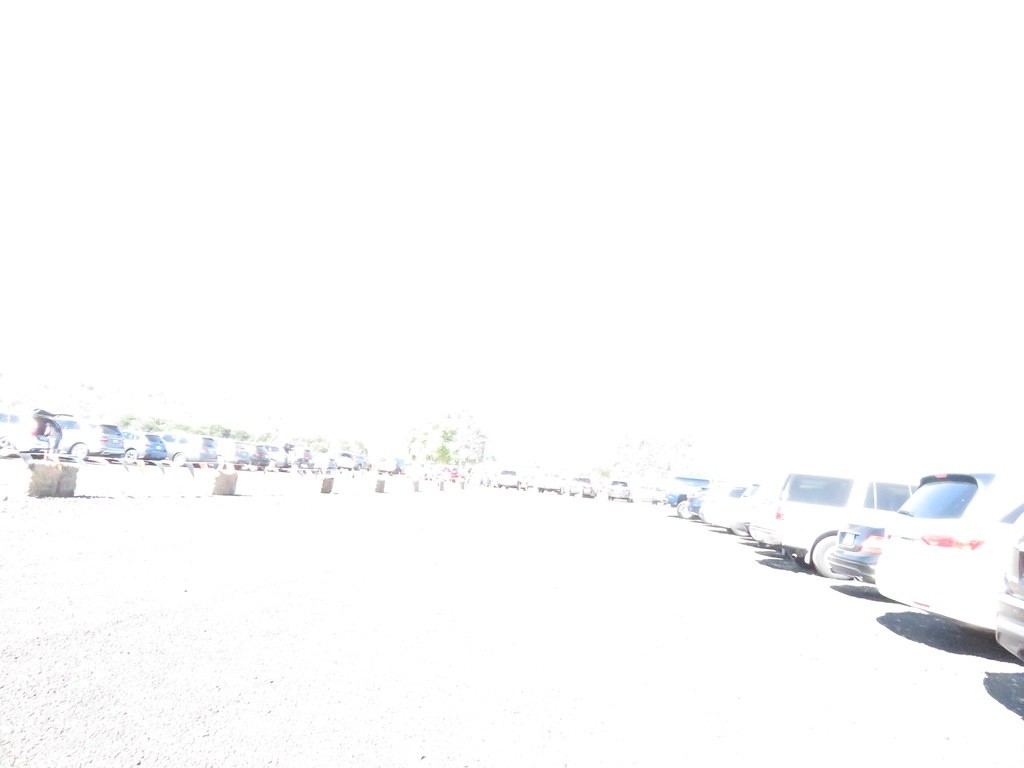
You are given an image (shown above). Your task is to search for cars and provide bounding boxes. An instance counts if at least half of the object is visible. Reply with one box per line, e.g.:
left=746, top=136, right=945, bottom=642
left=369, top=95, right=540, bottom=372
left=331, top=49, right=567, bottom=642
left=0, top=406, right=373, bottom=472
left=496, top=468, right=530, bottom=490
left=534, top=472, right=567, bottom=494
left=606, top=479, right=633, bottom=501
left=378, top=457, right=405, bottom=476
left=568, top=476, right=599, bottom=497
left=641, top=468, right=1024, bottom=663
